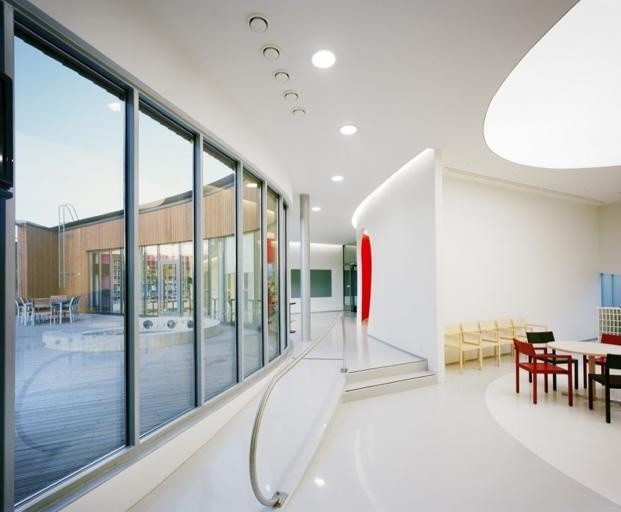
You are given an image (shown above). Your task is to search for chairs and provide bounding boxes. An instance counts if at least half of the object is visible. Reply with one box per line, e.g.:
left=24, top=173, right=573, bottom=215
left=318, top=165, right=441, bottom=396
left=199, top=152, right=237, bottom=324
left=15, top=294, right=83, bottom=327
left=443, top=316, right=621, bottom=423
